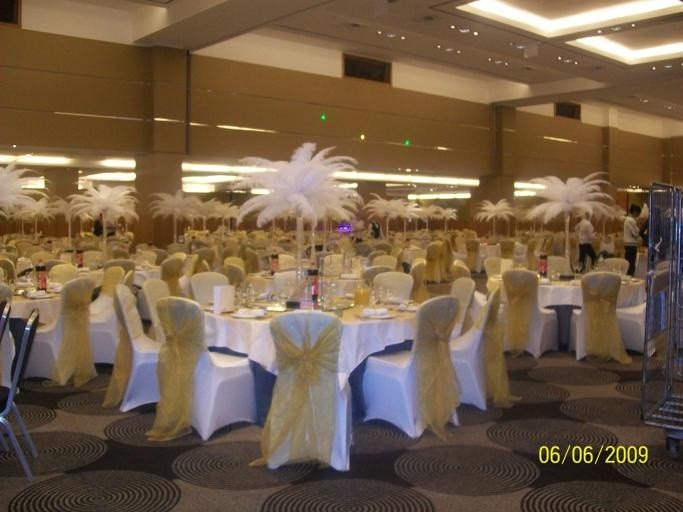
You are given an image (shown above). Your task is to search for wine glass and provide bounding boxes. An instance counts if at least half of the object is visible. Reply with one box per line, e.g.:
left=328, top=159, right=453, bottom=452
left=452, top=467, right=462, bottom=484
left=548, top=260, right=640, bottom=285
left=341, top=258, right=352, bottom=275
left=212, top=263, right=420, bottom=320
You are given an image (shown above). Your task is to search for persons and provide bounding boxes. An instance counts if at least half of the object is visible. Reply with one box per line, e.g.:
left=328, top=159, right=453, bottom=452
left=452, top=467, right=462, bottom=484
left=575, top=212, right=596, bottom=272
left=639, top=208, right=668, bottom=261
left=623, top=203, right=641, bottom=277
left=366, top=216, right=385, bottom=240
left=92, top=213, right=116, bottom=237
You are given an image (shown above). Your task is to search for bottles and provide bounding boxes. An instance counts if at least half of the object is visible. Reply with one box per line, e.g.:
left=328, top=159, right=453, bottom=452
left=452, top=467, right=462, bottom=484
left=75, top=244, right=84, bottom=268
left=270, top=249, right=280, bottom=277
left=35, top=259, right=49, bottom=291
left=538, top=250, right=548, bottom=278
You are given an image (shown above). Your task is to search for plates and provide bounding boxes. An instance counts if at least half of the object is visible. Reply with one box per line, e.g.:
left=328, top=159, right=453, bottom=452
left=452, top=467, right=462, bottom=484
left=9, top=277, right=63, bottom=300
left=89, top=258, right=160, bottom=273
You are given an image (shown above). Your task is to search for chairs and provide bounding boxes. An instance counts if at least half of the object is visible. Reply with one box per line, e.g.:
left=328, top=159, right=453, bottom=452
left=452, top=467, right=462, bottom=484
left=1, top=228, right=677, bottom=479
